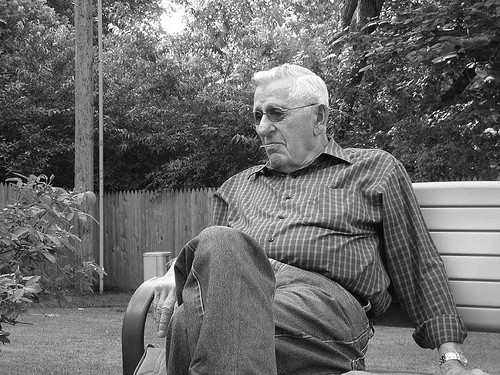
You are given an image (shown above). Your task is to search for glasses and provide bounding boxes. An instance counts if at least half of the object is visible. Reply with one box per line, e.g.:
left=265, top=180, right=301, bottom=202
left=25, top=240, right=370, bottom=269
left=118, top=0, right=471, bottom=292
left=247, top=103, right=321, bottom=127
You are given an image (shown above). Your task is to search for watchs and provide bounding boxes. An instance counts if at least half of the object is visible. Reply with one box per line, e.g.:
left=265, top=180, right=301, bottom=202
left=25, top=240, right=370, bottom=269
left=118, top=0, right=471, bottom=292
left=438, top=351, right=469, bottom=367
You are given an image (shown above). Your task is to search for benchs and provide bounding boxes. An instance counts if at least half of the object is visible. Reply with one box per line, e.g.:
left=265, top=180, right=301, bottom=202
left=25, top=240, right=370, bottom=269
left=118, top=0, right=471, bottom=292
left=122, top=180, right=500, bottom=375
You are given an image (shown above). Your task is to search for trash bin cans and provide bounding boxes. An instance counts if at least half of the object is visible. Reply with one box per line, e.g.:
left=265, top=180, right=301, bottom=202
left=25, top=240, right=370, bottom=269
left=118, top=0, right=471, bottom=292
left=142, top=252, right=171, bottom=282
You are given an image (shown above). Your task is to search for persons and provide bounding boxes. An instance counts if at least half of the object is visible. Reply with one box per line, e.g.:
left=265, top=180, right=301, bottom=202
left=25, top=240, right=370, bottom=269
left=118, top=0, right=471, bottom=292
left=152, top=63, right=494, bottom=375
